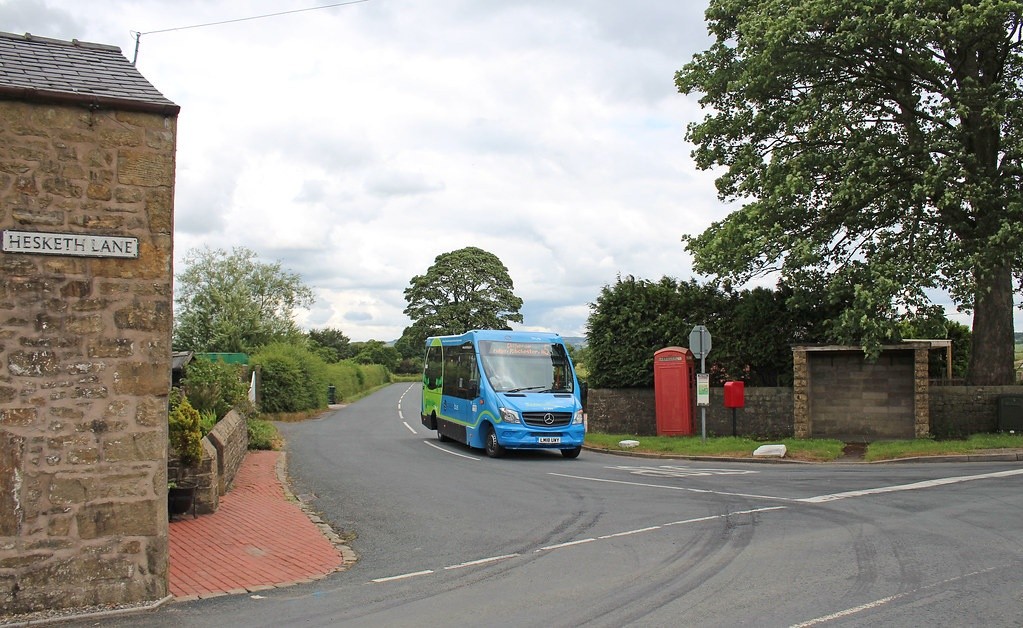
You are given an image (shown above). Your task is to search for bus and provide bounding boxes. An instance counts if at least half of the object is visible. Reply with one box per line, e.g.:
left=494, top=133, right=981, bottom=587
left=420, top=330, right=588, bottom=460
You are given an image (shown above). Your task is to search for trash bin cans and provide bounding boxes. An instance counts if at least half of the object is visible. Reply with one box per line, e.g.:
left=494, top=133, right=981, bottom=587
left=328, top=385, right=336, bottom=404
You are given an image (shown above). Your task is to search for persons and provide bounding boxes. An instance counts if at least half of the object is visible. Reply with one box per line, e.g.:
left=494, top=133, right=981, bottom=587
left=490, top=363, right=517, bottom=389
